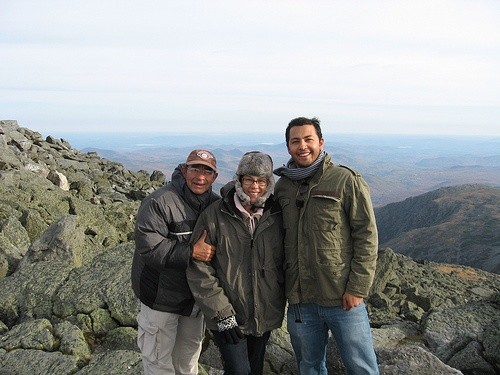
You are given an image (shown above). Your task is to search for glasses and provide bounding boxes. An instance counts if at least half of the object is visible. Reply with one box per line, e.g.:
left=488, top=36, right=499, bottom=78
left=187, top=168, right=213, bottom=176
left=295, top=181, right=309, bottom=208
left=243, top=176, right=269, bottom=186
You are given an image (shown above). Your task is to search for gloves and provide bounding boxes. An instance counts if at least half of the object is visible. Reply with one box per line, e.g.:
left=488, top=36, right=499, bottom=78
left=216, top=316, right=244, bottom=345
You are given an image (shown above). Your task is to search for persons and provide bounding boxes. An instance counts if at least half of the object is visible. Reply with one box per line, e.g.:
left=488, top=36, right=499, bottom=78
left=132, top=149, right=222, bottom=375
left=273, top=117, right=380, bottom=375
left=185, top=151, right=287, bottom=375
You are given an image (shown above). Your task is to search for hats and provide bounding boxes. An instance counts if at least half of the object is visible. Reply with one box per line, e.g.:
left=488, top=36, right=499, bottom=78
left=233, top=150, right=274, bottom=212
left=186, top=149, right=216, bottom=173
